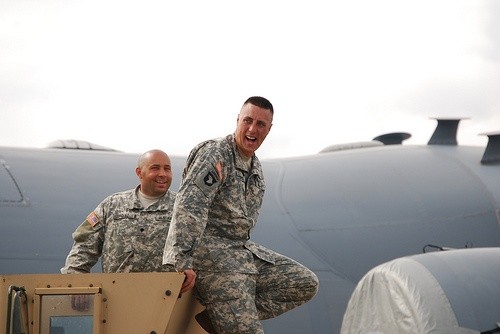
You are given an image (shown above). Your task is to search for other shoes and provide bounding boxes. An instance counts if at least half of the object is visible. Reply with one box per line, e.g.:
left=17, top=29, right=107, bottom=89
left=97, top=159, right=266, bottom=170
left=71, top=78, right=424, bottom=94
left=195, top=309, right=218, bottom=334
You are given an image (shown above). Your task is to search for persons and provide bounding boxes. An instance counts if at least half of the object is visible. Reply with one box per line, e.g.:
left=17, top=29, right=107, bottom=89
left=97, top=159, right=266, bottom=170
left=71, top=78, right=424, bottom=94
left=162, top=96, right=320, bottom=334
left=60, top=149, right=177, bottom=312
